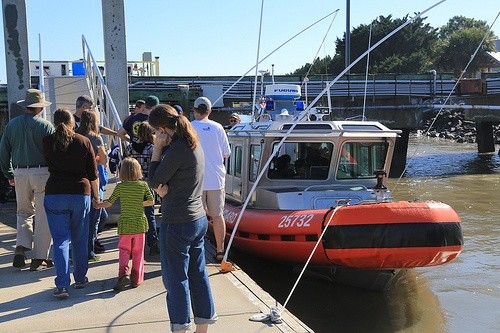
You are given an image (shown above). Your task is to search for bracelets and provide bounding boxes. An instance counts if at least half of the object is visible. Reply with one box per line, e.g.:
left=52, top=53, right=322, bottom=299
left=93, top=195, right=100, bottom=200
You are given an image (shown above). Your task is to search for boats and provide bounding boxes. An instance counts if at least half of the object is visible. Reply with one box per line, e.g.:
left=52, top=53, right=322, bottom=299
left=207, top=0, right=463, bottom=294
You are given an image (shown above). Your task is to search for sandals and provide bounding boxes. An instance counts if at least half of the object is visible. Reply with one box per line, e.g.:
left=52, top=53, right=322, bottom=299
left=215, top=251, right=224, bottom=261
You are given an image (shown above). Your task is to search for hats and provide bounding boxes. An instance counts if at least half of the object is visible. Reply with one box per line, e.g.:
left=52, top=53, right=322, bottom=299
left=146, top=95, right=159, bottom=105
left=173, top=105, right=183, bottom=112
left=17, top=88, right=53, bottom=107
left=193, top=96, right=211, bottom=109
left=230, top=113, right=241, bottom=120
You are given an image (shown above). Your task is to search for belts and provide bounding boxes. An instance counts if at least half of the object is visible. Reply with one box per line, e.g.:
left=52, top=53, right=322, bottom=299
left=14, top=165, right=50, bottom=169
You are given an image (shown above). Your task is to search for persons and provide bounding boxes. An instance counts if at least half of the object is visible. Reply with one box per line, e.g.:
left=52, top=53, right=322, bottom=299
left=93, top=157, right=155, bottom=290
left=148, top=104, right=217, bottom=333
left=44, top=108, right=102, bottom=298
left=71, top=94, right=184, bottom=260
left=268, top=141, right=330, bottom=179
left=189, top=96, right=232, bottom=260
left=0, top=88, right=56, bottom=270
left=224, top=112, right=241, bottom=132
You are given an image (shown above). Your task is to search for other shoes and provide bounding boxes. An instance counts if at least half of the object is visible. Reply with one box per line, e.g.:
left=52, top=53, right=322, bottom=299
left=74, top=277, right=88, bottom=288
left=87, top=255, right=101, bottom=262
left=95, top=243, right=105, bottom=251
left=30, top=258, right=53, bottom=270
left=13, top=255, right=32, bottom=267
left=113, top=275, right=130, bottom=291
left=54, top=287, right=69, bottom=297
left=131, top=283, right=139, bottom=287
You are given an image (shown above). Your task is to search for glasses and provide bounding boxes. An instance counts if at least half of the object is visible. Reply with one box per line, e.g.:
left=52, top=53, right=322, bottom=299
left=230, top=119, right=238, bottom=122
